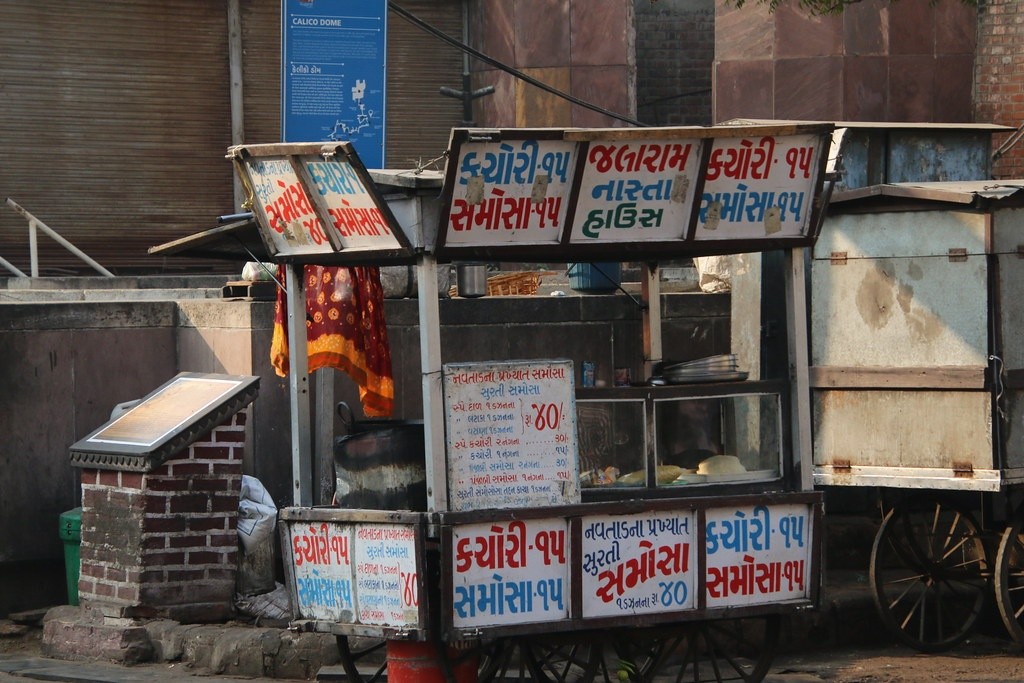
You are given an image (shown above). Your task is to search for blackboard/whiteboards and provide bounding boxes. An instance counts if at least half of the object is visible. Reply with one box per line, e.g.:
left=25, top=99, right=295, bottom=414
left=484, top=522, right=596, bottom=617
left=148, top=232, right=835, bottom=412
left=443, top=360, right=581, bottom=504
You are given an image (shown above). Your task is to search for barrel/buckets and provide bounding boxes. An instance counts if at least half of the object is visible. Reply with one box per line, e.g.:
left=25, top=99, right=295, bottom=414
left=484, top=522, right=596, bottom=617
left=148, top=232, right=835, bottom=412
left=567, top=263, right=620, bottom=295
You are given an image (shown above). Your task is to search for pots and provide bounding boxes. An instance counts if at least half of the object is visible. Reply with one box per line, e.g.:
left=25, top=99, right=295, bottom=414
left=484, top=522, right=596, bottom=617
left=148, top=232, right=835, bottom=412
left=335, top=402, right=424, bottom=436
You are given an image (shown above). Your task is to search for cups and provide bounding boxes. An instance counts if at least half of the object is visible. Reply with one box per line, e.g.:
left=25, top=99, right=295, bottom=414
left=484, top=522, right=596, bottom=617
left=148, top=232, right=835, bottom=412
left=615, top=367, right=631, bottom=387
left=456, top=264, right=487, bottom=295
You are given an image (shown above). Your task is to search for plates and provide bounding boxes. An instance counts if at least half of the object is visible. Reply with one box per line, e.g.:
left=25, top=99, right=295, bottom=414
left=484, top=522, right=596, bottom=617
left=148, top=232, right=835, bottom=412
left=658, top=354, right=749, bottom=384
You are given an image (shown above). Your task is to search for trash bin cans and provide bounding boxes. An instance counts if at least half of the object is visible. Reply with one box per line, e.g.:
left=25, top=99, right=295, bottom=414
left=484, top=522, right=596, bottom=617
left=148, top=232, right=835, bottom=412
left=58, top=508, right=81, bottom=606
left=366, top=169, right=451, bottom=299
left=567, top=260, right=623, bottom=296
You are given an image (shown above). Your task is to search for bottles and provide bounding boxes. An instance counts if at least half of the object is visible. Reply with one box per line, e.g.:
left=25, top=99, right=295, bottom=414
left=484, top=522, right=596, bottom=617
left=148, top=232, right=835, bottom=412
left=581, top=351, right=598, bottom=386
